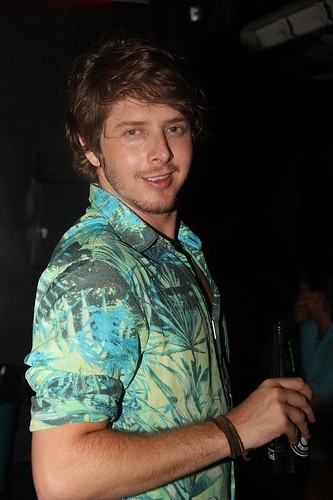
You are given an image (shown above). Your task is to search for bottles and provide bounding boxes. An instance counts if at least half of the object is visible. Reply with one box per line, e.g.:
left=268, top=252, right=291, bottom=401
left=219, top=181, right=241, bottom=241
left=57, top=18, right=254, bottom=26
left=267, top=321, right=309, bottom=476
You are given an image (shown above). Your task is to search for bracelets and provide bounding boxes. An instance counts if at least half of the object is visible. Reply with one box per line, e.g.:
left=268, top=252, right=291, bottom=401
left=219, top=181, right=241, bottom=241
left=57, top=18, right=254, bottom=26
left=204, top=413, right=248, bottom=461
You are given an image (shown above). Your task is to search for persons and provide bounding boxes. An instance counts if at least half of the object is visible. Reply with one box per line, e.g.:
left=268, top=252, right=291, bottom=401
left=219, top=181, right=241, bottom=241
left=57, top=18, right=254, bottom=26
left=23, top=42, right=315, bottom=500
left=293, top=264, right=333, bottom=500
